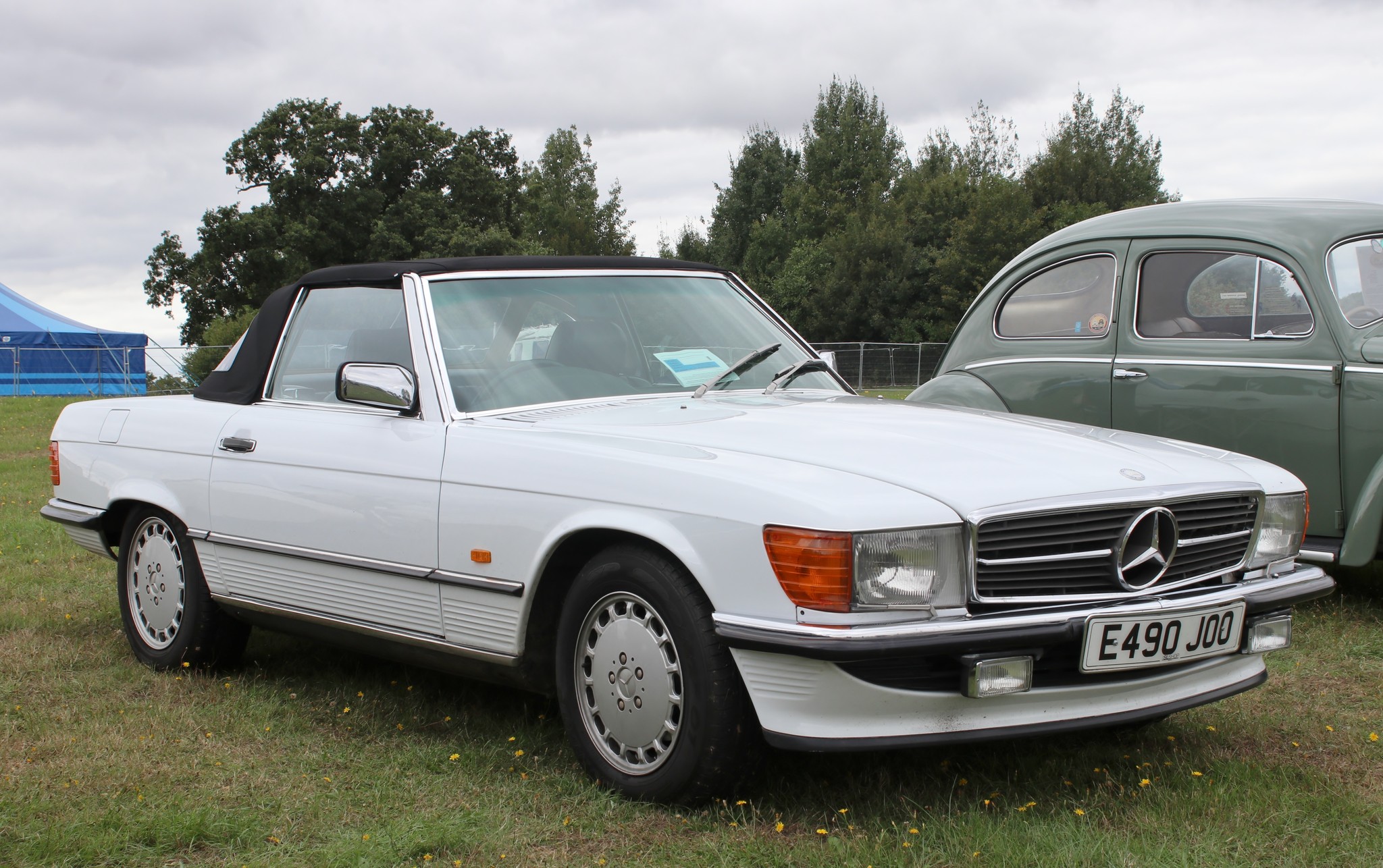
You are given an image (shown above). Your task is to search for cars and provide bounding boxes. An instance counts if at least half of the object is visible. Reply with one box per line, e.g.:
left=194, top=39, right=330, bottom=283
left=40, top=255, right=1334, bottom=807
left=902, top=199, right=1381, bottom=585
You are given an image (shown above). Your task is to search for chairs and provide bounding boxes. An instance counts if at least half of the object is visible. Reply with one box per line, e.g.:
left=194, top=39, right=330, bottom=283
left=325, top=326, right=470, bottom=415
left=546, top=320, right=654, bottom=394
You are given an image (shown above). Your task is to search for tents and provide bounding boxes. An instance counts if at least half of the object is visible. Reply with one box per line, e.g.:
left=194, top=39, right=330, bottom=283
left=0, top=280, right=150, bottom=398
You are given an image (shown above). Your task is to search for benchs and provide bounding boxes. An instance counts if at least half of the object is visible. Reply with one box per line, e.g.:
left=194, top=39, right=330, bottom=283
left=1271, top=320, right=1313, bottom=335
left=1138, top=316, right=1205, bottom=336
